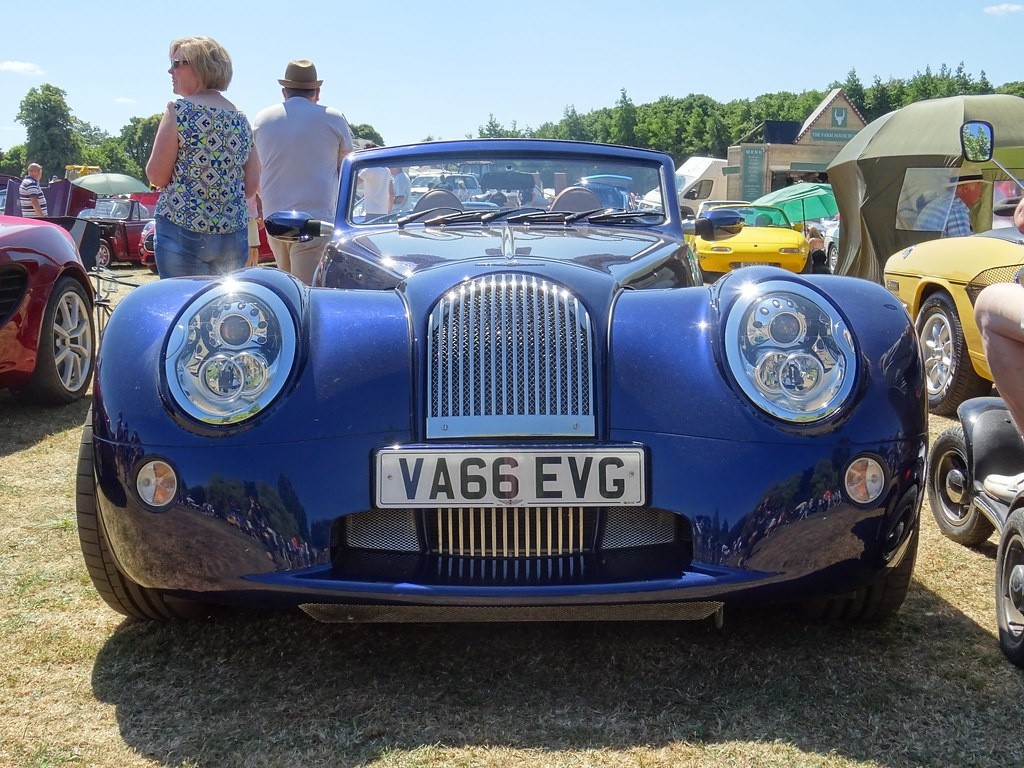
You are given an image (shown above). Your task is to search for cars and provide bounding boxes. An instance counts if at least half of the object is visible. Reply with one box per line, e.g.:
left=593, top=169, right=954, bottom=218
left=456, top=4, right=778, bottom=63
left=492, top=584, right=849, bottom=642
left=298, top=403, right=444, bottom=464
left=76, top=139, right=928, bottom=626
left=684, top=201, right=811, bottom=283
left=0, top=166, right=276, bottom=408
left=354, top=162, right=632, bottom=224
left=884, top=227, right=1023, bottom=416
left=824, top=228, right=839, bottom=274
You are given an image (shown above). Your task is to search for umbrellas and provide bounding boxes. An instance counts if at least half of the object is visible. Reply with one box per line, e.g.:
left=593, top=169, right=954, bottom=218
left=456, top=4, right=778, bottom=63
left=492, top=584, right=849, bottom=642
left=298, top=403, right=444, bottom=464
left=71, top=173, right=152, bottom=193
left=738, top=181, right=840, bottom=236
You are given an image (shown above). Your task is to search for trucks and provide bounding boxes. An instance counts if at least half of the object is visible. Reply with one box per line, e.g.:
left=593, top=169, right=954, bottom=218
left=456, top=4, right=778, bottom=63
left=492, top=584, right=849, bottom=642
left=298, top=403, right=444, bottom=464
left=638, top=156, right=727, bottom=220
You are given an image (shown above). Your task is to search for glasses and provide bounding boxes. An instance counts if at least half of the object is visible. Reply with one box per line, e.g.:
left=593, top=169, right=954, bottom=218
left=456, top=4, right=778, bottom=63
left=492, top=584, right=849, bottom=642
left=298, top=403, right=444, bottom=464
left=170, top=58, right=190, bottom=69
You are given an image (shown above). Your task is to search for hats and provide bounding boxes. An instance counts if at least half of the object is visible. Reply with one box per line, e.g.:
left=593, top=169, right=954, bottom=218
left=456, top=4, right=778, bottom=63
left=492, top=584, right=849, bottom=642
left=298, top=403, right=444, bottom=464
left=941, top=166, right=993, bottom=189
left=279, top=60, right=323, bottom=89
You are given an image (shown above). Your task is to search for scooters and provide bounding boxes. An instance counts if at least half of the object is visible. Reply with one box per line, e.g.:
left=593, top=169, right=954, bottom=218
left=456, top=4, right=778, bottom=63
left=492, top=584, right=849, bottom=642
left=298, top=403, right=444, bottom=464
left=927, top=121, right=1024, bottom=667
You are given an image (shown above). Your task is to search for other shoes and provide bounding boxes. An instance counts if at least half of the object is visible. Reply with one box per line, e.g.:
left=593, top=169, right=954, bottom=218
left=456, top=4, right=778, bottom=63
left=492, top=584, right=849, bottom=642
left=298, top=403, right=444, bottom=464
left=983, top=473, right=1024, bottom=504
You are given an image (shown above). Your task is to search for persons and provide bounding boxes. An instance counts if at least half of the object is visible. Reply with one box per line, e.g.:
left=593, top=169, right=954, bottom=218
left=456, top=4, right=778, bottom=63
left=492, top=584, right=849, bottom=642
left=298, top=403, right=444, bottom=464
left=357, top=142, right=412, bottom=223
left=915, top=159, right=994, bottom=239
left=808, top=226, right=832, bottom=275
left=428, top=175, right=454, bottom=191
left=146, top=35, right=261, bottom=280
left=19, top=162, right=48, bottom=217
left=458, top=181, right=468, bottom=201
left=974, top=197, right=1024, bottom=506
left=251, top=60, right=353, bottom=286
left=489, top=189, right=506, bottom=206
left=246, top=194, right=260, bottom=267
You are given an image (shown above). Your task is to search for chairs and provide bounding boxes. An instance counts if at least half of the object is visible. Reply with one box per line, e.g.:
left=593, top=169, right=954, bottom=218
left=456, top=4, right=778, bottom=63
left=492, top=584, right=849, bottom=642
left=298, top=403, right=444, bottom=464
left=412, top=189, right=464, bottom=222
left=753, top=213, right=774, bottom=228
left=550, top=186, right=605, bottom=220
